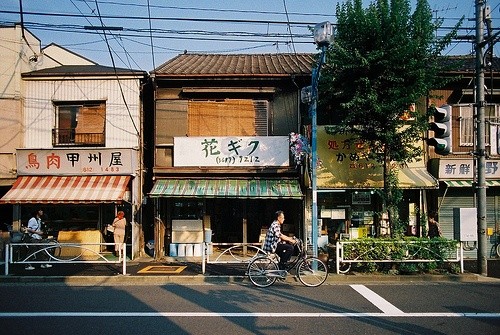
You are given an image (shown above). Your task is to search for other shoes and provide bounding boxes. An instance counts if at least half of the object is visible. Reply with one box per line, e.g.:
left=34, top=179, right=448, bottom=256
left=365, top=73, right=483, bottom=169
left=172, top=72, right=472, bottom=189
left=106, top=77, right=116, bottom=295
left=25, top=266, right=35, bottom=270
left=40, top=264, right=52, bottom=268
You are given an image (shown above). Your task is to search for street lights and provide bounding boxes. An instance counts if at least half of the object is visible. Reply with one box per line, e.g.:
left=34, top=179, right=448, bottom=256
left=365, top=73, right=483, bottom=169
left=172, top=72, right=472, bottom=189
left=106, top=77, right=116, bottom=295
left=300, top=21, right=335, bottom=273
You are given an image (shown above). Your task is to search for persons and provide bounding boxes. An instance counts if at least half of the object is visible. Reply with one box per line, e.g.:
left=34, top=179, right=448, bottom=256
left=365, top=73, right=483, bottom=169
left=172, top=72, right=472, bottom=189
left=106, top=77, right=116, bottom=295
left=428, top=214, right=443, bottom=236
left=112, top=212, right=130, bottom=257
left=262, top=210, right=295, bottom=284
left=26, top=209, right=53, bottom=270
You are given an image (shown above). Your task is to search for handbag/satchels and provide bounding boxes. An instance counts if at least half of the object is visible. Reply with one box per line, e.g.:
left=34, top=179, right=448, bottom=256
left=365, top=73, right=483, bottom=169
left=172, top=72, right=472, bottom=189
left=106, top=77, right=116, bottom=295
left=21, top=233, right=30, bottom=243
left=107, top=224, right=114, bottom=233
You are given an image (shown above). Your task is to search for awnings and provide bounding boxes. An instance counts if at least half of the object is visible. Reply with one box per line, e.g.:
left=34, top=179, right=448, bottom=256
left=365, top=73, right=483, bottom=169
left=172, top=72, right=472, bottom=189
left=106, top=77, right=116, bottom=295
left=443, top=180, right=500, bottom=187
left=304, top=168, right=440, bottom=193
left=0, top=175, right=135, bottom=205
left=147, top=179, right=305, bottom=200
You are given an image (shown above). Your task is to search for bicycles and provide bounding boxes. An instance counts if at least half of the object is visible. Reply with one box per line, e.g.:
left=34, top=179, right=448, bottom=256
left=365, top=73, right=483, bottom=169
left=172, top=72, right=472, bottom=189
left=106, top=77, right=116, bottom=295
left=11, top=224, right=62, bottom=267
left=247, top=236, right=328, bottom=288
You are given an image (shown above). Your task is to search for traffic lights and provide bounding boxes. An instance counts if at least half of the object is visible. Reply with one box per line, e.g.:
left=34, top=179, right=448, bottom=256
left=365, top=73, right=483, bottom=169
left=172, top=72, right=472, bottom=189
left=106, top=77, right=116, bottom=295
left=426, top=105, right=452, bottom=156
